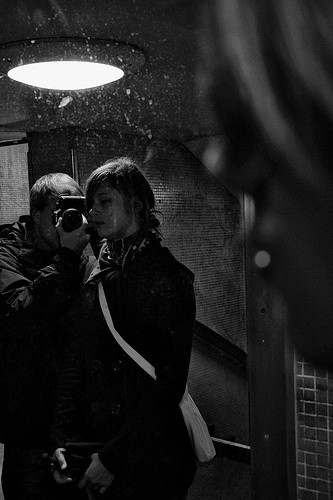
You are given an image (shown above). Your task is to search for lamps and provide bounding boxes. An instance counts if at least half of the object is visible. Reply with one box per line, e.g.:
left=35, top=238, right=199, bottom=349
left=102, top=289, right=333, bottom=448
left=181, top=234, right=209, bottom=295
left=7, top=0, right=127, bottom=92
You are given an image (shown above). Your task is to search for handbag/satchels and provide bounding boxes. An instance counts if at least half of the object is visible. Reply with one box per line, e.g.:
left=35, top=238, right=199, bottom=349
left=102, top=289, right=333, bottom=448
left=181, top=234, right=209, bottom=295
left=168, top=384, right=216, bottom=464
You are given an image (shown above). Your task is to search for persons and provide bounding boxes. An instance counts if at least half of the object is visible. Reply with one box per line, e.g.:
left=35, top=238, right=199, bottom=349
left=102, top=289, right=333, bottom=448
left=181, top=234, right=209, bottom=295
left=44, top=154, right=200, bottom=500
left=0, top=172, right=109, bottom=500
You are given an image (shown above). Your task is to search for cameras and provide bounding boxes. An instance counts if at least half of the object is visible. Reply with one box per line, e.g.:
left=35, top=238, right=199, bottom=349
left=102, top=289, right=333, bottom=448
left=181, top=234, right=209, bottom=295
left=53, top=195, right=88, bottom=234
